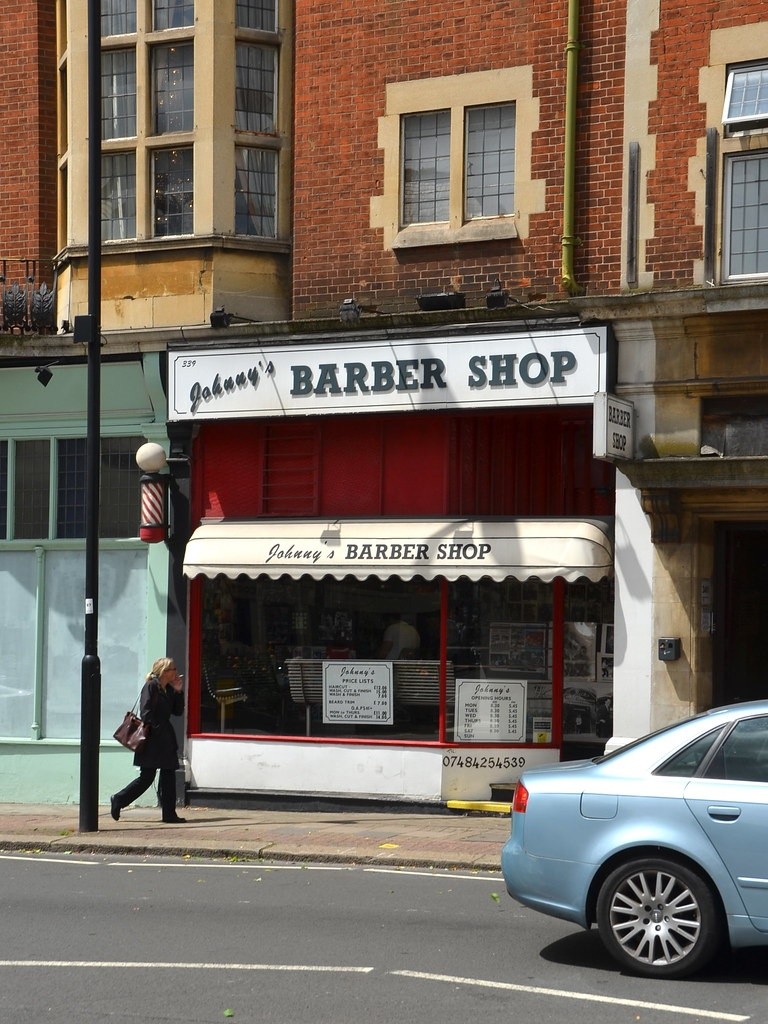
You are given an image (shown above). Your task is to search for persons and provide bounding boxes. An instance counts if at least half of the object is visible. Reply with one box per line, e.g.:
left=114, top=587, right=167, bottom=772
left=382, top=616, right=421, bottom=659
left=326, top=632, right=350, bottom=660
left=111, top=657, right=185, bottom=823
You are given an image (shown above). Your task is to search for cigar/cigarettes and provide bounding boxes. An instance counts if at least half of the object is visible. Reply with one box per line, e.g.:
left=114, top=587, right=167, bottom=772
left=178, top=674, right=183, bottom=678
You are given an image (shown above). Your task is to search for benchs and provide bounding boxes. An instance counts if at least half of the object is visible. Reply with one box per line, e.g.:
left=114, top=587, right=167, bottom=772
left=284, top=659, right=456, bottom=737
left=203, top=663, right=248, bottom=734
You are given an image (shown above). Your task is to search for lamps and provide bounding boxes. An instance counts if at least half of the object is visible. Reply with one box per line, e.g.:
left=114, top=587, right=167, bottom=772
left=339, top=299, right=387, bottom=324
left=486, top=278, right=523, bottom=310
left=35, top=361, right=59, bottom=387
left=210, top=307, right=262, bottom=328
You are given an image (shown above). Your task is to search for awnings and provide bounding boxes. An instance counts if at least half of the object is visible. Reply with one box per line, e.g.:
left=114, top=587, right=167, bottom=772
left=183, top=515, right=616, bottom=583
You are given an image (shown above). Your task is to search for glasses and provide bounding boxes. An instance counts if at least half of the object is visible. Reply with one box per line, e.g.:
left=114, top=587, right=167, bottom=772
left=164, top=668, right=177, bottom=673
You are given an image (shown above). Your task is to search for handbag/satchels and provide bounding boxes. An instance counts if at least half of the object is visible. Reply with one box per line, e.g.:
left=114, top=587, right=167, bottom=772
left=113, top=712, right=152, bottom=753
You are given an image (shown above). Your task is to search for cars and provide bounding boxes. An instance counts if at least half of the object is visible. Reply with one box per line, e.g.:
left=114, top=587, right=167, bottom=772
left=499, top=695, right=768, bottom=981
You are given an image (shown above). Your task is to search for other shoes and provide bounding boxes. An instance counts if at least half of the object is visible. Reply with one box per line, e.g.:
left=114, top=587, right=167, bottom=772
left=162, top=815, right=187, bottom=824
left=110, top=795, right=121, bottom=821
